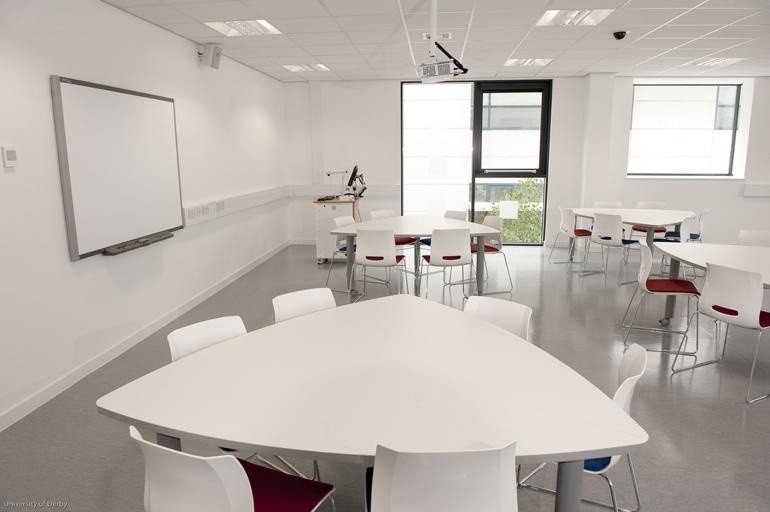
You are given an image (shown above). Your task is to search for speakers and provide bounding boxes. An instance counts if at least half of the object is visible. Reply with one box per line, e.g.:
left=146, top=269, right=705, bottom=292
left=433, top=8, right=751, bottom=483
left=202, top=47, right=223, bottom=70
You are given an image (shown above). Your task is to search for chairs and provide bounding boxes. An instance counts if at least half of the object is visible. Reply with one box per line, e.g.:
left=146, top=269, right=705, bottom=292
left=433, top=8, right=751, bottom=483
left=166, top=315, right=316, bottom=480
left=671, top=262, right=770, bottom=403
left=548, top=200, right=710, bottom=286
left=363, top=441, right=518, bottom=512
left=272, top=287, right=337, bottom=323
left=518, top=343, right=648, bottom=512
left=621, top=239, right=701, bottom=355
left=736, top=230, right=770, bottom=248
left=324, top=210, right=513, bottom=304
left=128, top=425, right=337, bottom=512
left=462, top=297, right=533, bottom=487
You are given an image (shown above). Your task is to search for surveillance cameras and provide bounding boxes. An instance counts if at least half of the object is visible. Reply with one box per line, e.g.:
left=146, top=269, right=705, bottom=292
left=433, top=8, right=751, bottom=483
left=612, top=31, right=628, bottom=41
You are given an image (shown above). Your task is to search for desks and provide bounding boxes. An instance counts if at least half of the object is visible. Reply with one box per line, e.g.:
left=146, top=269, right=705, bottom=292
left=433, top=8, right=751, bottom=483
left=96, top=294, right=650, bottom=512
left=653, top=242, right=770, bottom=325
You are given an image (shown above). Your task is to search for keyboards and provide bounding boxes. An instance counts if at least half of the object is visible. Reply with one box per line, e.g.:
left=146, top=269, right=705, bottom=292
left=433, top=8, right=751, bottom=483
left=317, top=195, right=334, bottom=203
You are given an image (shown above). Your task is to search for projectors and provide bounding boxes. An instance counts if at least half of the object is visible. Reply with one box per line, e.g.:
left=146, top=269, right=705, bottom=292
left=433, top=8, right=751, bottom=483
left=417, top=58, right=456, bottom=86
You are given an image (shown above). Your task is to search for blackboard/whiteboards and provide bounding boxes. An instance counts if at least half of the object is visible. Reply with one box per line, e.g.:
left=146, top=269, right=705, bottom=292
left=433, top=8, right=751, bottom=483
left=48, top=71, right=185, bottom=262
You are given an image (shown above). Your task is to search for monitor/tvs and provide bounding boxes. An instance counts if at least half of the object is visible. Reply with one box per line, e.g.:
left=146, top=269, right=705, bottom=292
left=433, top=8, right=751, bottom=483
left=348, top=164, right=358, bottom=187
